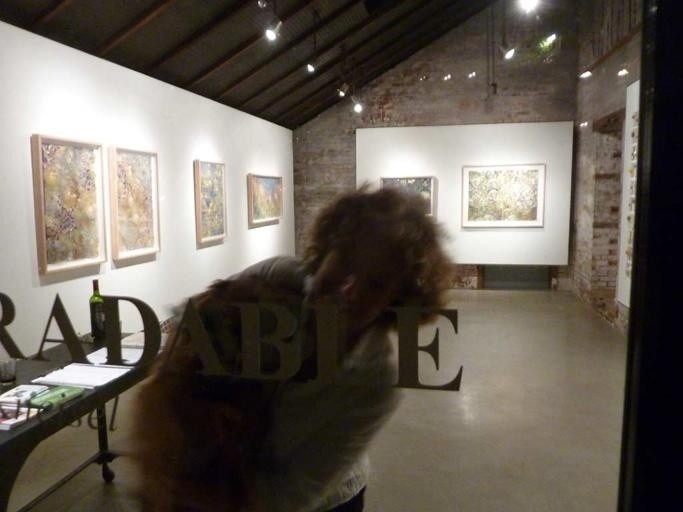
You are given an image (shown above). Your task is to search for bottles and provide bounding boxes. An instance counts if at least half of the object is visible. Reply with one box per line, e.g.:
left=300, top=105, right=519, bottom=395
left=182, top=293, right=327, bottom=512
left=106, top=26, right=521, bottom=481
left=88, top=278, right=106, bottom=341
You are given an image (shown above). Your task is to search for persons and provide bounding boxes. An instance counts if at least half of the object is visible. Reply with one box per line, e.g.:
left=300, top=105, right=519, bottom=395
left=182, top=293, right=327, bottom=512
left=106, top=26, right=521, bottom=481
left=133, top=182, right=463, bottom=512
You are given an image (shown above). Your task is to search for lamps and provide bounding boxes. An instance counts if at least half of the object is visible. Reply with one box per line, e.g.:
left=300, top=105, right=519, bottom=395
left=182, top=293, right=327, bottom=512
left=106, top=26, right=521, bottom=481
left=498, top=42, right=515, bottom=62
left=264, top=19, right=281, bottom=42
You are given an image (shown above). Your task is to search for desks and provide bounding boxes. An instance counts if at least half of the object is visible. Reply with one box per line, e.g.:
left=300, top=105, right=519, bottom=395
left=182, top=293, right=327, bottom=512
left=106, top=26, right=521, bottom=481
left=0, top=332, right=169, bottom=512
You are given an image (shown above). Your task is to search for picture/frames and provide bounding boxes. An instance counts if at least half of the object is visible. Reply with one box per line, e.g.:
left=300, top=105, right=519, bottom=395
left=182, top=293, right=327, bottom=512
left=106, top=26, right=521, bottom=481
left=460, top=163, right=547, bottom=230
left=245, top=174, right=283, bottom=229
left=378, top=176, right=435, bottom=217
left=27, top=132, right=159, bottom=285
left=193, top=159, right=228, bottom=246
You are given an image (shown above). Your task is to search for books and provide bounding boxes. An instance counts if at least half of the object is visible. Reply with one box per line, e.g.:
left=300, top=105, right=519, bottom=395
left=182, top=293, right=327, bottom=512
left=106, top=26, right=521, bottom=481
left=26, top=385, right=84, bottom=411
left=0, top=403, right=43, bottom=433
left=0, top=382, right=49, bottom=407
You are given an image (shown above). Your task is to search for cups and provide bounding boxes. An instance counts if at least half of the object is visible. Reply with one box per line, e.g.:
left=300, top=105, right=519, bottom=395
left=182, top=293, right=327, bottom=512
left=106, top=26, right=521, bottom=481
left=0, top=358, right=19, bottom=387
